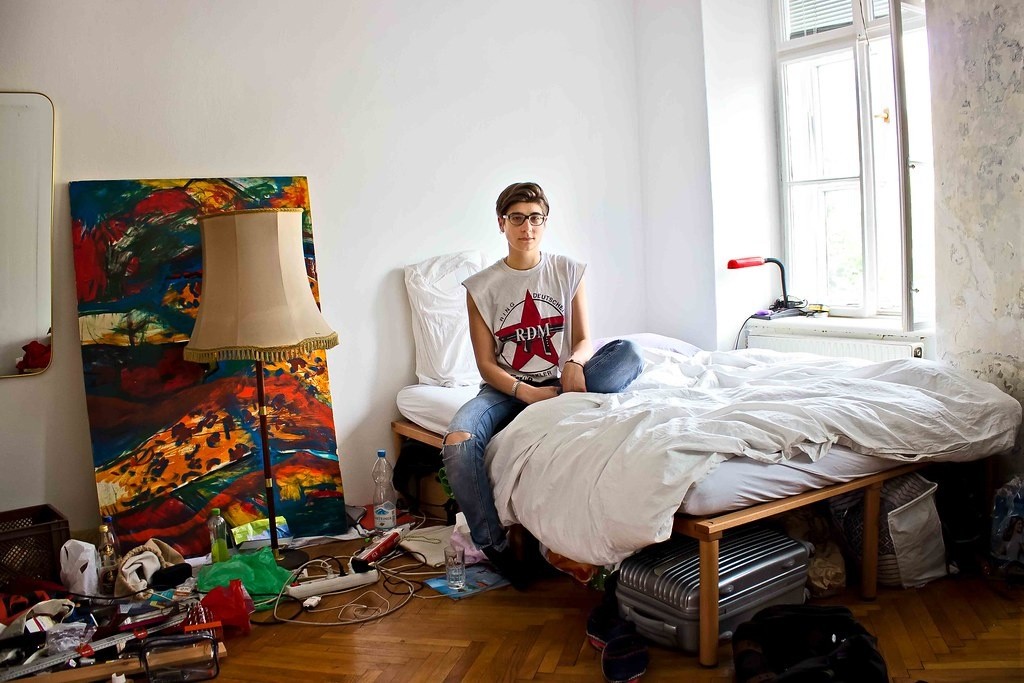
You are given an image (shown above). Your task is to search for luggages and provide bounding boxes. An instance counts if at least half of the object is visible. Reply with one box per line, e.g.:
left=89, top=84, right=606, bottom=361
left=615, top=522, right=810, bottom=655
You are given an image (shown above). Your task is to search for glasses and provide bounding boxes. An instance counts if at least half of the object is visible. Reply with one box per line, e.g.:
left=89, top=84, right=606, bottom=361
left=502, top=212, right=547, bottom=226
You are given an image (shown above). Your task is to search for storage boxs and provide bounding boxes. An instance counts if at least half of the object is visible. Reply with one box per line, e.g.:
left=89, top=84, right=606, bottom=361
left=0, top=503, right=72, bottom=597
left=417, top=472, right=459, bottom=526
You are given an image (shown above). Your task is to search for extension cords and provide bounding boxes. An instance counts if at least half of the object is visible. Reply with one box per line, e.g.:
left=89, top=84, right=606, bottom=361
left=285, top=568, right=378, bottom=600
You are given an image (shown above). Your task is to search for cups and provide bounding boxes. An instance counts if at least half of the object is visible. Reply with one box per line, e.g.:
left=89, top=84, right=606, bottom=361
left=444, top=546, right=466, bottom=589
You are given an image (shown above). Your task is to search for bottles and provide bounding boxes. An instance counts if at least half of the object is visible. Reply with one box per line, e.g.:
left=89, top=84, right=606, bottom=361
left=372, top=449, right=397, bottom=532
left=98, top=526, right=115, bottom=567
left=207, top=508, right=228, bottom=564
left=101, top=516, right=121, bottom=561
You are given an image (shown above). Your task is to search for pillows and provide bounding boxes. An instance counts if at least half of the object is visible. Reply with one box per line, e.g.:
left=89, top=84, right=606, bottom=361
left=592, top=329, right=701, bottom=374
left=401, top=250, right=486, bottom=389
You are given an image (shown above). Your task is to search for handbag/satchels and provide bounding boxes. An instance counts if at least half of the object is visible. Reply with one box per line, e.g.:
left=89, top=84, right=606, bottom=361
left=0, top=598, right=75, bottom=665
left=60, top=539, right=99, bottom=602
left=0, top=590, right=50, bottom=627
left=731, top=603, right=889, bottom=683
left=831, top=471, right=949, bottom=588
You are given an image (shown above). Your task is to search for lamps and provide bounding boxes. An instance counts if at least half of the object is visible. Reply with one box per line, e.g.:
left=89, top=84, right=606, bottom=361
left=729, top=255, right=803, bottom=321
left=183, top=208, right=339, bottom=571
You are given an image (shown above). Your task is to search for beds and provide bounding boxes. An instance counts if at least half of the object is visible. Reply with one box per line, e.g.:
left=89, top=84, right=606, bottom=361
left=389, top=351, right=1024, bottom=672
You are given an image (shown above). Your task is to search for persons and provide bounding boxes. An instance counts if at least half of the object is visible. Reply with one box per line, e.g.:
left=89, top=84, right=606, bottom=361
left=439, top=183, right=644, bottom=593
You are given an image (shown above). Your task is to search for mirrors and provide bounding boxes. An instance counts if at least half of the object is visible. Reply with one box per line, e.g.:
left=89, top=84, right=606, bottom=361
left=0, top=90, right=56, bottom=379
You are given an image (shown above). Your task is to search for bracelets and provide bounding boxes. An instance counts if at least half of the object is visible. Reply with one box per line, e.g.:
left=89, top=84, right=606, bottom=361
left=564, top=359, right=584, bottom=368
left=511, top=380, right=521, bottom=396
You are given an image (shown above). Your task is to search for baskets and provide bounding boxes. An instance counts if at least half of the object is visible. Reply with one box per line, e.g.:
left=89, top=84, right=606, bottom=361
left=0, top=504, right=70, bottom=595
